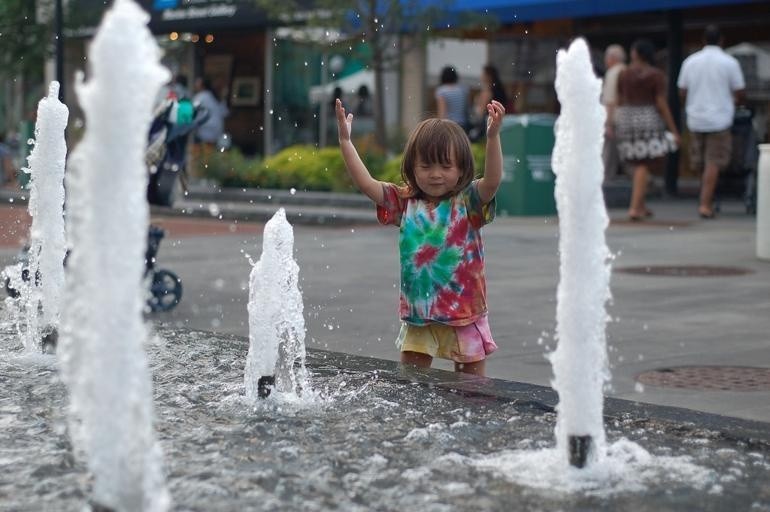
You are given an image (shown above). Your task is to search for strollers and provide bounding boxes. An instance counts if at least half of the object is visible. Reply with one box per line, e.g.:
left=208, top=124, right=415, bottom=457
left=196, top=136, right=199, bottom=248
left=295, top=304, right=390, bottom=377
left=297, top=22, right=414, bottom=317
left=5, top=99, right=210, bottom=313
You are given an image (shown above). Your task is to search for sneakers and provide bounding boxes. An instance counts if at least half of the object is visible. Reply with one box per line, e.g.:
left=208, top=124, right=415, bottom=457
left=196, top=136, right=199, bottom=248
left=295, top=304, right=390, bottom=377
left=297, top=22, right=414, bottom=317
left=628, top=203, right=756, bottom=221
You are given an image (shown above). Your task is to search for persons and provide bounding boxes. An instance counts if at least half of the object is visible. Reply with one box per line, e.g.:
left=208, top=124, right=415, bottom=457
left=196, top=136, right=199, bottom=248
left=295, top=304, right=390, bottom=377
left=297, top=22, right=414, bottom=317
left=432, top=62, right=468, bottom=127
left=352, top=85, right=376, bottom=119
left=617, top=39, right=682, bottom=221
left=163, top=72, right=231, bottom=161
left=329, top=85, right=348, bottom=118
left=333, top=93, right=506, bottom=379
left=474, top=64, right=504, bottom=129
left=677, top=26, right=746, bottom=218
left=599, top=43, right=629, bottom=136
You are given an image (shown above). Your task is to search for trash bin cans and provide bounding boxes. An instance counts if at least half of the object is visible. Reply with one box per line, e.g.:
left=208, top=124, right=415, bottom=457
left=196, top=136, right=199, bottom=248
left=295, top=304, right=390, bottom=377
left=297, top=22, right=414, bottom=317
left=494, top=114, right=559, bottom=216
